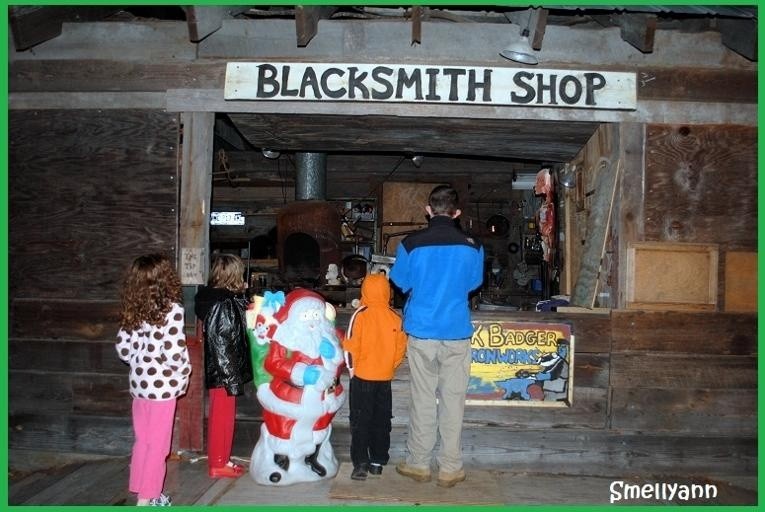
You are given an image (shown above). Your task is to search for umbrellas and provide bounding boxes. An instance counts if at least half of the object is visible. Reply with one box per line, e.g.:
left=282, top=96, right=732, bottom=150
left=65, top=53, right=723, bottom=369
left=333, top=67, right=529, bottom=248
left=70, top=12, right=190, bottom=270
left=209, top=461, right=244, bottom=477
left=396, top=460, right=466, bottom=487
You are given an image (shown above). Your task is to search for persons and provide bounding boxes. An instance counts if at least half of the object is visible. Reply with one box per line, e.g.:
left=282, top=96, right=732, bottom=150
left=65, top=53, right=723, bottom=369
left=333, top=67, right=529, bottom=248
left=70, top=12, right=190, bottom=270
left=341, top=272, right=407, bottom=480
left=114, top=251, right=193, bottom=506
left=194, top=254, right=253, bottom=477
left=389, top=185, right=485, bottom=488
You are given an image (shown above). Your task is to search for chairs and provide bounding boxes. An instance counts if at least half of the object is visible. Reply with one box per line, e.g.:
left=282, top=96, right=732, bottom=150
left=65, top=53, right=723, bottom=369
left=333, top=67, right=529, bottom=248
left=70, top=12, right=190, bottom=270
left=136, top=493, right=172, bottom=506
left=351, top=463, right=382, bottom=480
